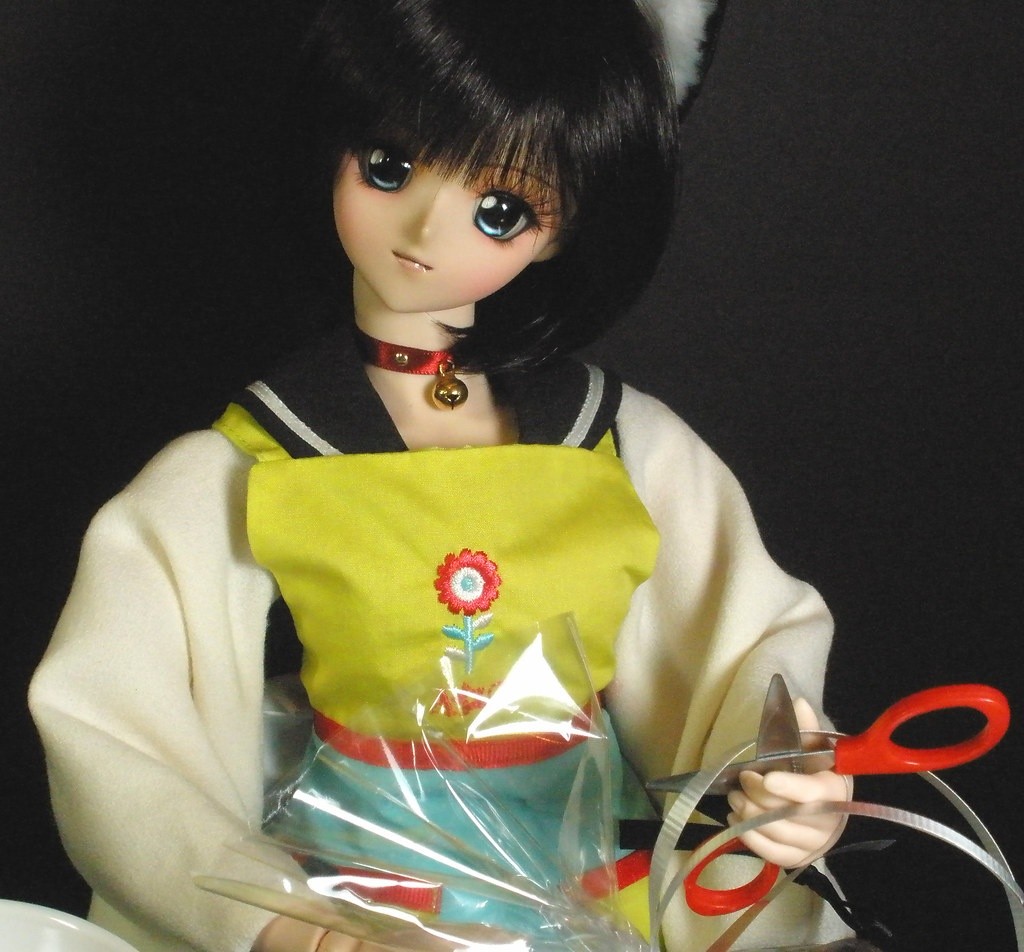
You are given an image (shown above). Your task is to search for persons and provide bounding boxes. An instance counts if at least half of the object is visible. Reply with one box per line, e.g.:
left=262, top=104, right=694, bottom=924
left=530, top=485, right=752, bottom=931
left=29, top=0, right=856, bottom=952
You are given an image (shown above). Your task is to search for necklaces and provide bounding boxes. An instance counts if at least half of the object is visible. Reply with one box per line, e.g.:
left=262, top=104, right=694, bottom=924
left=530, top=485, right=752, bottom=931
left=353, top=326, right=468, bottom=409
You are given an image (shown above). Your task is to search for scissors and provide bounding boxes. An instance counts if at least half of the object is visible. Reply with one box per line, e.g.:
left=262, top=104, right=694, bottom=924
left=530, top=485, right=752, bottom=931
left=645, top=673, right=1011, bottom=919
